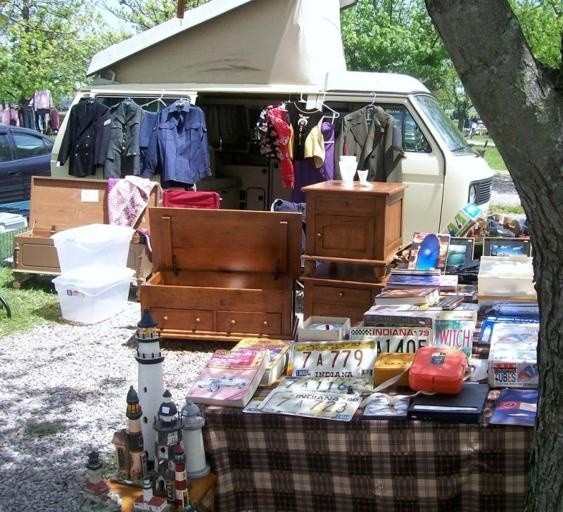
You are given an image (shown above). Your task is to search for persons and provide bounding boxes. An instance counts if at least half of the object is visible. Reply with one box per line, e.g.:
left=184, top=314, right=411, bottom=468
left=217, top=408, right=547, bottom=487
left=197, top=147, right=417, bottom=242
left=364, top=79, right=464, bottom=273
left=470, top=116, right=478, bottom=128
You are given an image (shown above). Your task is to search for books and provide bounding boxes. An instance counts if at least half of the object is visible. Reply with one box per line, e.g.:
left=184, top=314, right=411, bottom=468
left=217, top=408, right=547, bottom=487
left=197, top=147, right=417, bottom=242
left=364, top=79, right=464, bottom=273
left=186, top=349, right=270, bottom=407
left=364, top=230, right=541, bottom=363
left=231, top=337, right=295, bottom=387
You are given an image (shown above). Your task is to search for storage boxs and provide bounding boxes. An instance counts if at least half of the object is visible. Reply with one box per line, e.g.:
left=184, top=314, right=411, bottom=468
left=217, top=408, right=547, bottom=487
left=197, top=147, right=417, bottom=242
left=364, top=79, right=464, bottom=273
left=0, top=209, right=28, bottom=259
left=50, top=223, right=137, bottom=280
left=51, top=267, right=137, bottom=325
left=0, top=201, right=33, bottom=222
left=141, top=207, right=302, bottom=347
left=158, top=188, right=220, bottom=212
left=12, top=173, right=164, bottom=303
left=299, top=263, right=387, bottom=330
left=298, top=180, right=408, bottom=280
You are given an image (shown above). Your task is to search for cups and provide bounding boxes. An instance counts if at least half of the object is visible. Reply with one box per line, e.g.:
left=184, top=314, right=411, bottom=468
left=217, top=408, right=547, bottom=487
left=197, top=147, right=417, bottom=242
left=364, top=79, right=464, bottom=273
left=339, top=153, right=369, bottom=187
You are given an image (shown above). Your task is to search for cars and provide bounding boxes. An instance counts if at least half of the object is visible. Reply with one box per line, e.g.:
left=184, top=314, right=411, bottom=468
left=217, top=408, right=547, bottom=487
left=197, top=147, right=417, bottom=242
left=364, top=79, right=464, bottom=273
left=0, top=123, right=54, bottom=203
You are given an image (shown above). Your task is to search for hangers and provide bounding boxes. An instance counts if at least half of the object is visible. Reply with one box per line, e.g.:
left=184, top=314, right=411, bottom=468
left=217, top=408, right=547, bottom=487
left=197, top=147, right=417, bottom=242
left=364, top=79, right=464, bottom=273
left=80, top=88, right=379, bottom=120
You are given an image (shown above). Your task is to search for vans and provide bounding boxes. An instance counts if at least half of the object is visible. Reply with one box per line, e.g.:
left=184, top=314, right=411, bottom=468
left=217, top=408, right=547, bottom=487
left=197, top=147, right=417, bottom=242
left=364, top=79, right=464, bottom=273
left=50, top=0, right=493, bottom=246
